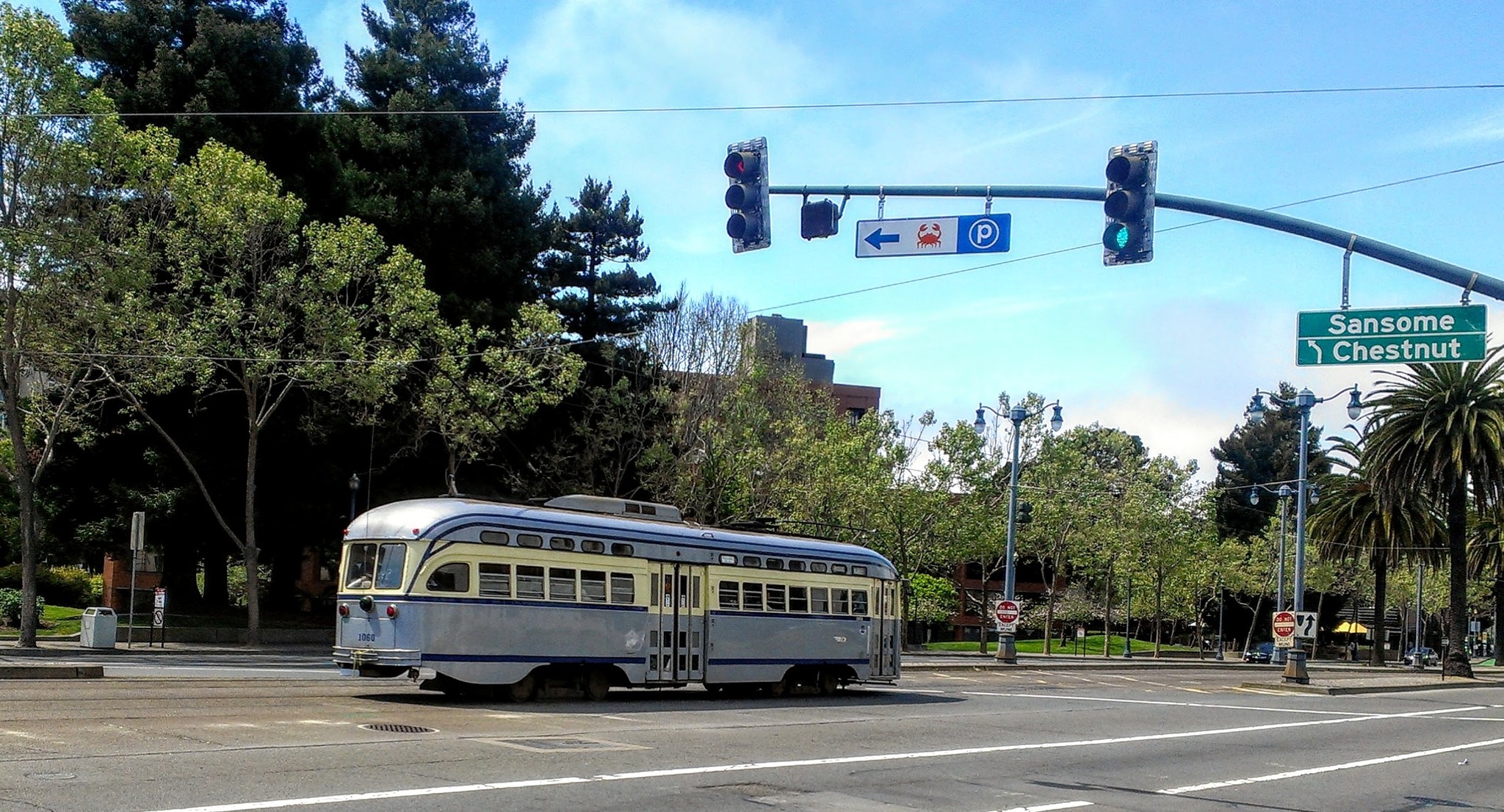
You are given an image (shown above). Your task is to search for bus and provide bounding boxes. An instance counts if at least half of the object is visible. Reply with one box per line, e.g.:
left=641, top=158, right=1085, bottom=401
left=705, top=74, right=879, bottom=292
left=333, top=335, right=915, bottom=704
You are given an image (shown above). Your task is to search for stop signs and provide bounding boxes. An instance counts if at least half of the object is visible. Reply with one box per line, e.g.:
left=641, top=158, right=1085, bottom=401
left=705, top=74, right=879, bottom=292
left=996, top=601, right=1018, bottom=623
left=1273, top=612, right=1295, bottom=637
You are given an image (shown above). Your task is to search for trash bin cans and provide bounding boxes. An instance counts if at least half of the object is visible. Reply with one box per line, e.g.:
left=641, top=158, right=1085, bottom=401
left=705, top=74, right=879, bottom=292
left=80, top=607, right=118, bottom=648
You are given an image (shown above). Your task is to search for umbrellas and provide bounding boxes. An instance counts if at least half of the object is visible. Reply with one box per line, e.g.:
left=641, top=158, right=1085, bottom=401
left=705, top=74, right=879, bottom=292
left=1331, top=622, right=1368, bottom=633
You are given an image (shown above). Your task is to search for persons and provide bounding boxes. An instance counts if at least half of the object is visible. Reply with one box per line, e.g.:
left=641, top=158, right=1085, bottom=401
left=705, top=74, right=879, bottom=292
left=1350, top=639, right=1358, bottom=661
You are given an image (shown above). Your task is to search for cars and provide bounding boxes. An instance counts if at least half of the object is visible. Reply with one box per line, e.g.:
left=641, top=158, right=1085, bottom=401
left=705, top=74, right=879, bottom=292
left=1404, top=647, right=1439, bottom=667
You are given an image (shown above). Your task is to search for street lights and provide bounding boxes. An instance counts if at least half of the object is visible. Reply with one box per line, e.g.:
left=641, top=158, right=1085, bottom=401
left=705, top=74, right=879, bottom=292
left=1468, top=607, right=1472, bottom=648
left=972, top=397, right=1064, bottom=664
left=1249, top=480, right=1320, bottom=665
left=1246, top=382, right=1363, bottom=685
left=1472, top=608, right=1478, bottom=658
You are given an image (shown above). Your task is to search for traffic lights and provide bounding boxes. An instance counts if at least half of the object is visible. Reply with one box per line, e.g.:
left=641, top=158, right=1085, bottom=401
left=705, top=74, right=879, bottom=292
left=1101, top=140, right=1158, bottom=267
left=724, top=136, right=771, bottom=253
